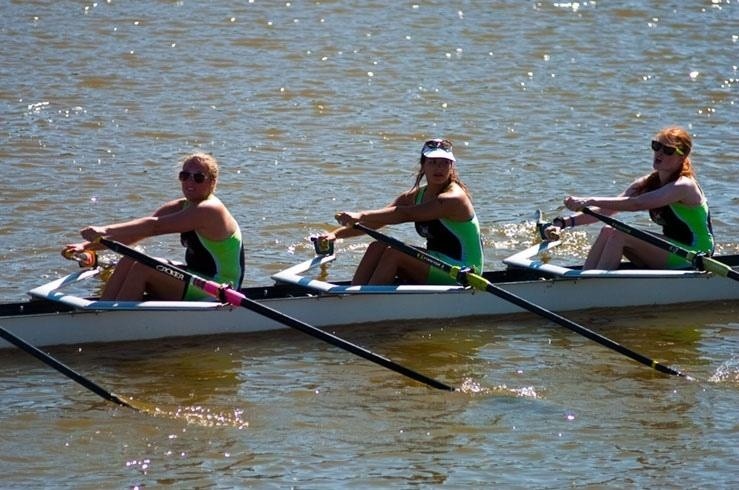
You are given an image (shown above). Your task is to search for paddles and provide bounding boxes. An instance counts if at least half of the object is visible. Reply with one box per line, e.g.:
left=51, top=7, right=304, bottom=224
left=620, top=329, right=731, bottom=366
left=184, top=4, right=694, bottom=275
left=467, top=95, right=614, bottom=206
left=535, top=209, right=560, bottom=240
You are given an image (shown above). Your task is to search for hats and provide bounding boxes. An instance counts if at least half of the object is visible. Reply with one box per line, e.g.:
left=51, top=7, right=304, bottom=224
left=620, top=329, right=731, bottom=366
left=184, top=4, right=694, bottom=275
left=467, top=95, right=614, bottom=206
left=421, top=138, right=458, bottom=164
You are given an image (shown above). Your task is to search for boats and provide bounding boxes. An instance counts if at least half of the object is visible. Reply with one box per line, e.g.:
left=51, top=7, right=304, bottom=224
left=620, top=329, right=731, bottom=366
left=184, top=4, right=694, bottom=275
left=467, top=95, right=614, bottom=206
left=0, top=236, right=738, bottom=354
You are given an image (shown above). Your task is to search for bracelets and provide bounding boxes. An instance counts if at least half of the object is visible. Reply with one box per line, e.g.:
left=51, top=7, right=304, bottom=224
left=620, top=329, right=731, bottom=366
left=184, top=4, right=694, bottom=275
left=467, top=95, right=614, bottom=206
left=568, top=213, right=577, bottom=228
left=551, top=216, right=567, bottom=230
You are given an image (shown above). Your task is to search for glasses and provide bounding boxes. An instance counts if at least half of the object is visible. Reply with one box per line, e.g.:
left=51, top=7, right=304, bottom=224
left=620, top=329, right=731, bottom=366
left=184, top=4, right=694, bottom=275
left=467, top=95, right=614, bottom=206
left=651, top=139, right=681, bottom=155
left=176, top=169, right=212, bottom=185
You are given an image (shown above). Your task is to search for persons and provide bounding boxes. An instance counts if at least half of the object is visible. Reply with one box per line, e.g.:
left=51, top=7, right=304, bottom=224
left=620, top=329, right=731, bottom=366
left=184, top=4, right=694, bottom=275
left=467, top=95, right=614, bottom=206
left=542, top=122, right=716, bottom=278
left=314, top=138, right=484, bottom=289
left=63, top=152, right=247, bottom=304
